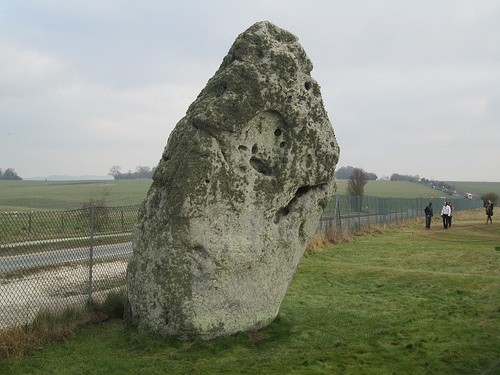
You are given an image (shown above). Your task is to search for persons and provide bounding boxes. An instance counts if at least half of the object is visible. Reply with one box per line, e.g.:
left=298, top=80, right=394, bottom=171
left=440, top=201, right=451, bottom=230
left=484, top=199, right=494, bottom=225
left=424, top=202, right=434, bottom=230
left=446, top=202, right=453, bottom=228
